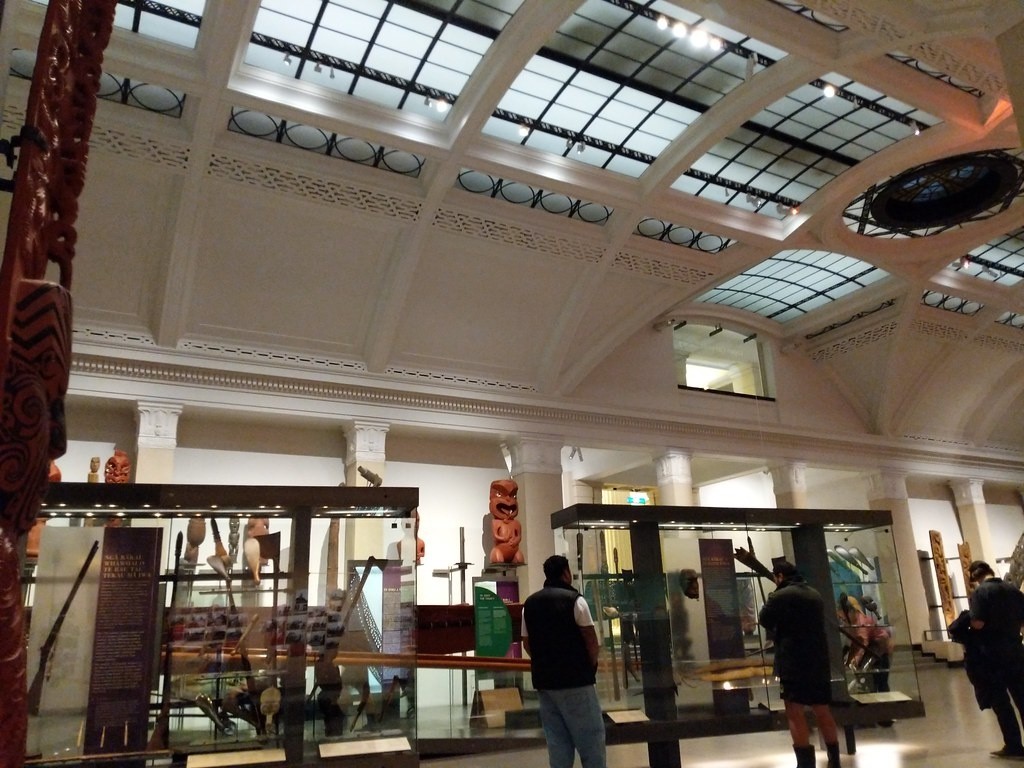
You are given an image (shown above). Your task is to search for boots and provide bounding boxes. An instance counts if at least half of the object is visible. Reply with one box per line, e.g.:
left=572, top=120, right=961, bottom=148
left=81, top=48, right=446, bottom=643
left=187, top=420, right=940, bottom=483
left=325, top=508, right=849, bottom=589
left=826, top=742, right=841, bottom=768
left=792, top=743, right=816, bottom=768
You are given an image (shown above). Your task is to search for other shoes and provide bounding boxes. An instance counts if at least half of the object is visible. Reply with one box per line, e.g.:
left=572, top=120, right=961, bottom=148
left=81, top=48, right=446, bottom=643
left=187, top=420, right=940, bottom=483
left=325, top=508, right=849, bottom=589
left=991, top=745, right=1024, bottom=759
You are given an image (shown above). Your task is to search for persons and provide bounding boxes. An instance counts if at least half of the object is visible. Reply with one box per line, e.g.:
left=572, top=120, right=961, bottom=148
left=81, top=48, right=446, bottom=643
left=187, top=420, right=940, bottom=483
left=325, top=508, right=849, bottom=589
left=489, top=480, right=524, bottom=564
left=839, top=595, right=893, bottom=693
left=521, top=555, right=606, bottom=768
left=758, top=561, right=841, bottom=768
left=968, top=561, right=1024, bottom=759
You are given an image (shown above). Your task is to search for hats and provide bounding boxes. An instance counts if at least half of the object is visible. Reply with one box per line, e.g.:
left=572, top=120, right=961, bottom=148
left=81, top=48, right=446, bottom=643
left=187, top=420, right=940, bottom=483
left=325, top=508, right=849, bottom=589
left=838, top=597, right=865, bottom=618
left=969, top=561, right=989, bottom=582
